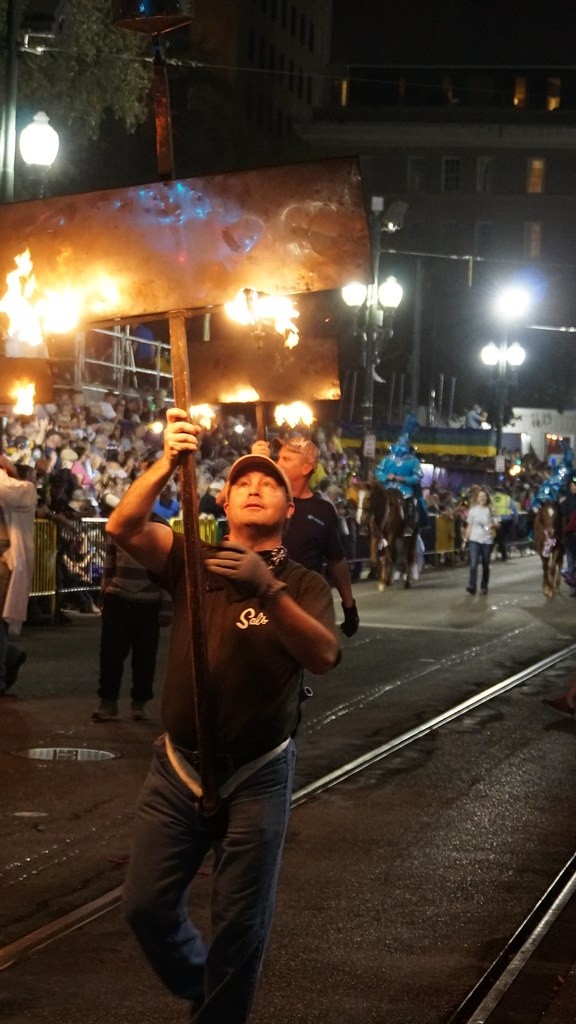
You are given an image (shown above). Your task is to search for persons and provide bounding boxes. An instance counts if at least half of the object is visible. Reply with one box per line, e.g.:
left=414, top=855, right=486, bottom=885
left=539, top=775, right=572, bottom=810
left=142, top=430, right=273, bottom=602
left=0, top=320, right=311, bottom=724
left=215, top=433, right=360, bottom=639
left=460, top=404, right=488, bottom=429
left=311, top=423, right=576, bottom=596
left=103, top=409, right=340, bottom=1024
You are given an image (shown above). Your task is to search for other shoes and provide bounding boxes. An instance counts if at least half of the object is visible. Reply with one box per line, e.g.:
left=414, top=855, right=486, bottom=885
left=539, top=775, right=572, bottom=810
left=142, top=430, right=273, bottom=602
left=128, top=700, right=145, bottom=720
left=466, top=587, right=476, bottom=596
left=92, top=700, right=119, bottom=719
left=0, top=645, right=26, bottom=694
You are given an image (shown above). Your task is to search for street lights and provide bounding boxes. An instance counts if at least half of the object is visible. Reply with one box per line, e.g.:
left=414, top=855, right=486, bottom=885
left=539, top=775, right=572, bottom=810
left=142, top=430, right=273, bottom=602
left=342, top=195, right=403, bottom=481
left=480, top=289, right=531, bottom=495
left=1, top=55, right=62, bottom=206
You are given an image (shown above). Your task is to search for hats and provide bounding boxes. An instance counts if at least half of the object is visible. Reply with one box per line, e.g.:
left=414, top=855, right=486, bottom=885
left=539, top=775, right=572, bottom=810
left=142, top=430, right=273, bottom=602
left=222, top=454, right=294, bottom=506
left=271, top=436, right=319, bottom=474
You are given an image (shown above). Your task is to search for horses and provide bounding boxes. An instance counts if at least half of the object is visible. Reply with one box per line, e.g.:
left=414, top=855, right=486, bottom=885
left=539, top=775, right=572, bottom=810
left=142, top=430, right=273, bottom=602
left=355, top=481, right=424, bottom=592
left=532, top=501, right=568, bottom=596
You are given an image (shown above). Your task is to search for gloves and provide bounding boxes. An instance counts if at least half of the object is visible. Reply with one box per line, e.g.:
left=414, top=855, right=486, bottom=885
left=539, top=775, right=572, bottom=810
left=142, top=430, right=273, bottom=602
left=340, top=599, right=360, bottom=638
